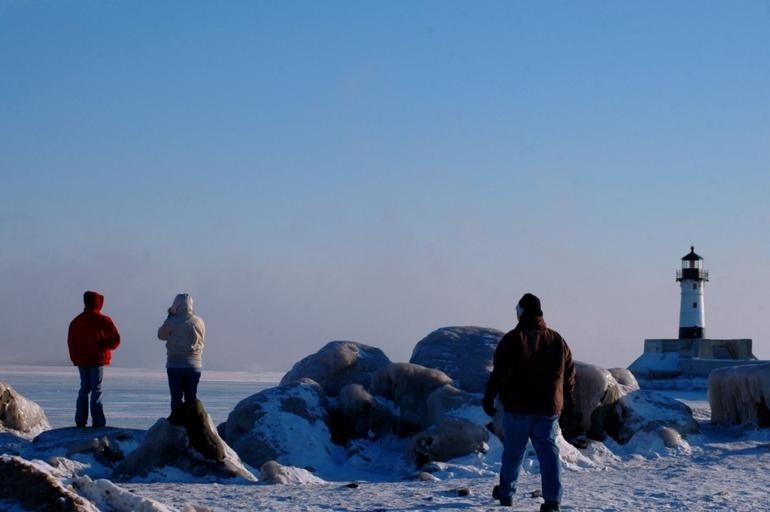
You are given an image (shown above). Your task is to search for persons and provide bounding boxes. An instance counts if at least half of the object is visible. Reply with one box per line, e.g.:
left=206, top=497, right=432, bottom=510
left=66, top=291, right=121, bottom=429
left=157, top=292, right=206, bottom=412
left=573, top=384, right=627, bottom=448
left=481, top=293, right=578, bottom=512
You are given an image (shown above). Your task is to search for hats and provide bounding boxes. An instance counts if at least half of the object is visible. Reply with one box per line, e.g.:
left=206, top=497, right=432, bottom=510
left=516, top=292, right=541, bottom=321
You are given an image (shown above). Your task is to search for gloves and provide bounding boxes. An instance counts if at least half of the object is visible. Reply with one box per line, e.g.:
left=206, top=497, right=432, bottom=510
left=564, top=399, right=576, bottom=412
left=482, top=394, right=498, bottom=417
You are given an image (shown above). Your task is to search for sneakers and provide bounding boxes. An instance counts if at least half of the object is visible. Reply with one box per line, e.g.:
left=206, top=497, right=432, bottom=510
left=540, top=503, right=560, bottom=512
left=492, top=485, right=513, bottom=506
